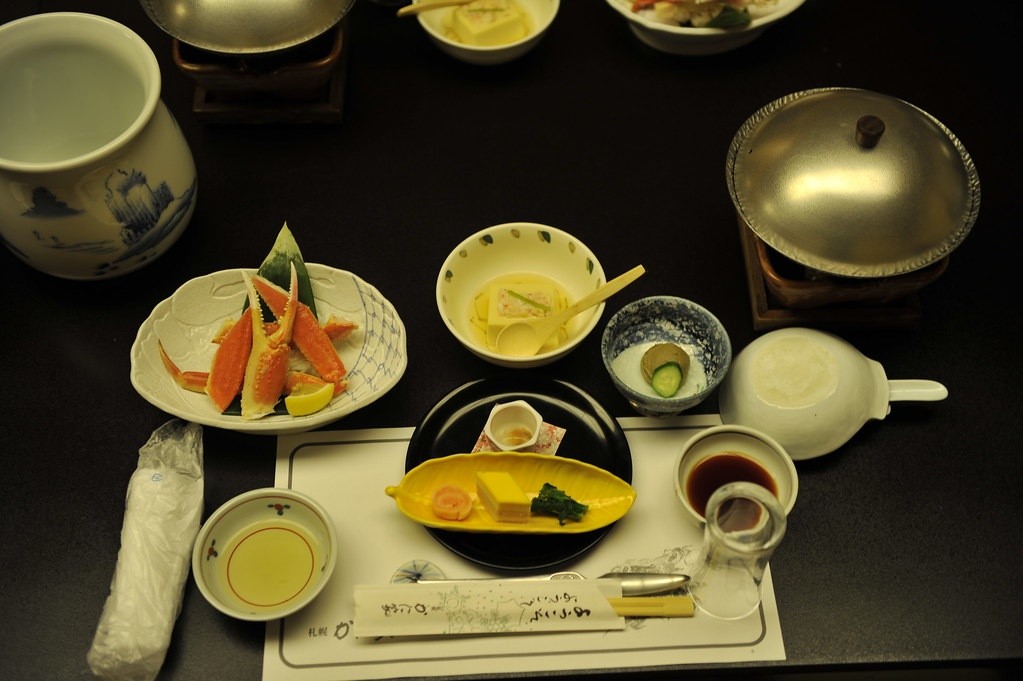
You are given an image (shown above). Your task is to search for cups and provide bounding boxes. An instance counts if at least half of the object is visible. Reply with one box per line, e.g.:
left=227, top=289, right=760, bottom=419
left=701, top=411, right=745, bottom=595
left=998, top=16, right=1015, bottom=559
left=0, top=12, right=197, bottom=280
left=688, top=482, right=786, bottom=621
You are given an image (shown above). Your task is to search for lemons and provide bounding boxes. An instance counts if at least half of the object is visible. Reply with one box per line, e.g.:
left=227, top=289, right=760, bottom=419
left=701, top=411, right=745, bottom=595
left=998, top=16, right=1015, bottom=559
left=285, top=382, right=335, bottom=417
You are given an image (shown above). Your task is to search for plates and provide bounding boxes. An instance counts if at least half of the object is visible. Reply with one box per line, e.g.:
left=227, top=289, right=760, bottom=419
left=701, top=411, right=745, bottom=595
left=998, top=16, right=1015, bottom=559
left=405, top=369, right=632, bottom=575
left=129, top=261, right=406, bottom=432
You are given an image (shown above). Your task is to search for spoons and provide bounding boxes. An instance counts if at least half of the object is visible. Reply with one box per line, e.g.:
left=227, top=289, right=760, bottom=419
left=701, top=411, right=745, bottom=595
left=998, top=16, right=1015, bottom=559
left=496, top=265, right=645, bottom=357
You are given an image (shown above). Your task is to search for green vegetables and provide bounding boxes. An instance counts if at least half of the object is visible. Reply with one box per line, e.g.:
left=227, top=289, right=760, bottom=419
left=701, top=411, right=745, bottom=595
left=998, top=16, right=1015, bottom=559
left=528, top=483, right=591, bottom=526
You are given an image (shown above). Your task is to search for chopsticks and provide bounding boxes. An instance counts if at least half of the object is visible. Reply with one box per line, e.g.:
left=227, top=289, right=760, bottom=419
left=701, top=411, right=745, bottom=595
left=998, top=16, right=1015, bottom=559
left=607, top=595, right=695, bottom=618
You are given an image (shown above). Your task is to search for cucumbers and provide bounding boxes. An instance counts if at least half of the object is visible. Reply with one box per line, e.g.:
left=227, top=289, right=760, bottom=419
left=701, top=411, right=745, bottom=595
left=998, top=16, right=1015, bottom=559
left=651, top=362, right=683, bottom=399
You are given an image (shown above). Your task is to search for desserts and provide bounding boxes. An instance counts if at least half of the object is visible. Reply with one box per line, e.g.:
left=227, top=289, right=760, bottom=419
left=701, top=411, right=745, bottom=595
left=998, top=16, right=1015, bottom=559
left=475, top=470, right=529, bottom=523
left=450, top=0, right=531, bottom=44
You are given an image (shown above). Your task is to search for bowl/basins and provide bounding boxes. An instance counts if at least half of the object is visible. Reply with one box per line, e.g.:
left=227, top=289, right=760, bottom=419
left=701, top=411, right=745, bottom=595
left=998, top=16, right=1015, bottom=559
left=754, top=236, right=952, bottom=312
left=192, top=488, right=338, bottom=620
left=606, top=0, right=806, bottom=55
left=483, top=400, right=543, bottom=451
left=436, top=222, right=607, bottom=365
left=674, top=423, right=799, bottom=527
left=413, top=0, right=560, bottom=65
left=602, top=296, right=731, bottom=411
left=172, top=25, right=343, bottom=98
left=717, top=327, right=889, bottom=460
